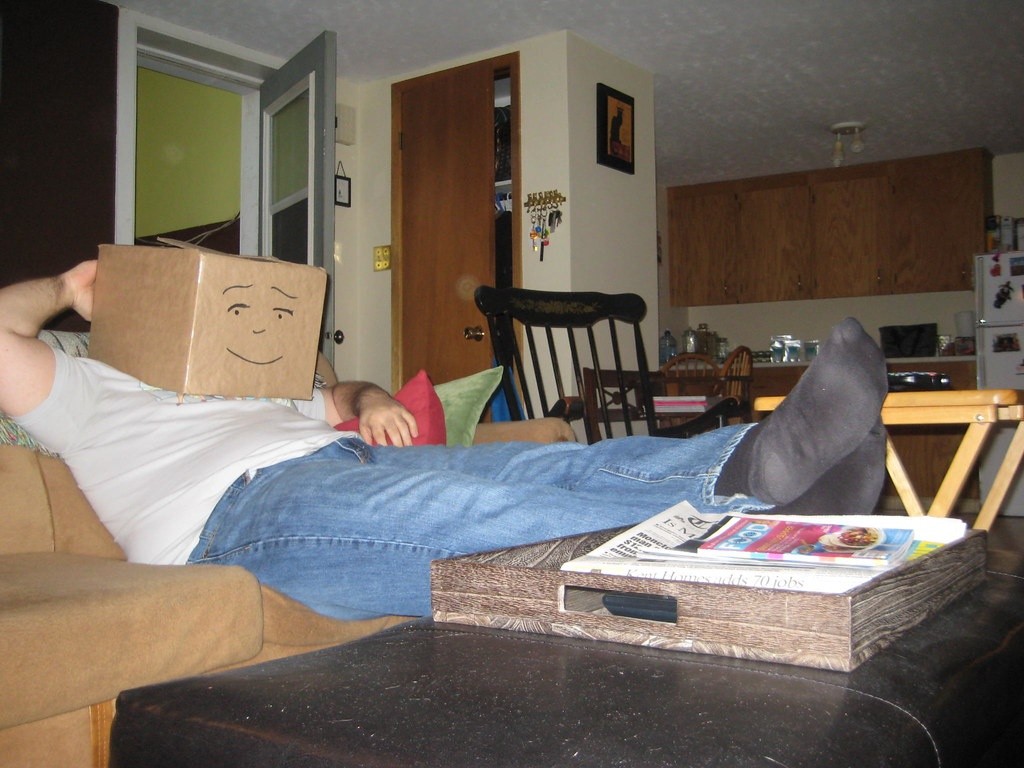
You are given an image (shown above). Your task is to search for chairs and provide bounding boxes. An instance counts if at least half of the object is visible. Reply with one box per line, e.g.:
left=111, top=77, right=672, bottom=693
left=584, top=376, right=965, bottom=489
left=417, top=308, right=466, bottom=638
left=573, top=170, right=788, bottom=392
left=476, top=285, right=752, bottom=455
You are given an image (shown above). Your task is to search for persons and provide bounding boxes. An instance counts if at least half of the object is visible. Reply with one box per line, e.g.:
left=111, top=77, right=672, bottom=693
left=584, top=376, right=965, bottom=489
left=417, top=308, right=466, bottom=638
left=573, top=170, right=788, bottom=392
left=2, top=253, right=892, bottom=626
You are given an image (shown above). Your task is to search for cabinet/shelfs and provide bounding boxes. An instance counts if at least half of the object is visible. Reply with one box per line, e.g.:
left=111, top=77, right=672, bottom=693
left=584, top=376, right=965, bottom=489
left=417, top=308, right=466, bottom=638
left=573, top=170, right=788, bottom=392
left=660, top=361, right=979, bottom=496
left=667, top=145, right=997, bottom=308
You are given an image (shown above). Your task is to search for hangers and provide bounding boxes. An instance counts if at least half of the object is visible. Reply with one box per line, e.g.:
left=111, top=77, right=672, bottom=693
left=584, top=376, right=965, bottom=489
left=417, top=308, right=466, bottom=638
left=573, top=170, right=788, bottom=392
left=493, top=191, right=514, bottom=219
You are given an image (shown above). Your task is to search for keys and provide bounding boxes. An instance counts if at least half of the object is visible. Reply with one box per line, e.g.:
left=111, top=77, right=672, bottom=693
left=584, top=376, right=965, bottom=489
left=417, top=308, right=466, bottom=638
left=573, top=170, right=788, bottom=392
left=555, top=209, right=562, bottom=226
left=529, top=230, right=538, bottom=251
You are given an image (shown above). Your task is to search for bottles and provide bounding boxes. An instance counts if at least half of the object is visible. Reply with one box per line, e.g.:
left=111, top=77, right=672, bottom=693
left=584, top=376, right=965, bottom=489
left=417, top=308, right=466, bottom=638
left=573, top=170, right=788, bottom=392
left=657, top=323, right=728, bottom=368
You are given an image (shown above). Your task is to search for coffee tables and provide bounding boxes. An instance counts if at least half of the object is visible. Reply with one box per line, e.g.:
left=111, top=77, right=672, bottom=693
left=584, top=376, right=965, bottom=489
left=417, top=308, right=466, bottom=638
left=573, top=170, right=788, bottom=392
left=109, top=532, right=1024, bottom=768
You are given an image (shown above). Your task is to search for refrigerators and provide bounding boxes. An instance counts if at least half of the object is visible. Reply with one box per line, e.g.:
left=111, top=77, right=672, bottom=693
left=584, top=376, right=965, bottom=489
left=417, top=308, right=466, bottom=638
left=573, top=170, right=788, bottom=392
left=970, top=251, right=1024, bottom=517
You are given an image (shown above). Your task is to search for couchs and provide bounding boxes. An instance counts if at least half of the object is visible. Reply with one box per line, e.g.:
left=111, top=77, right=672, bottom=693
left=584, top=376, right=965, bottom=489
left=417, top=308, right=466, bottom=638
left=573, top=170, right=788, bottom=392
left=0, top=329, right=582, bottom=767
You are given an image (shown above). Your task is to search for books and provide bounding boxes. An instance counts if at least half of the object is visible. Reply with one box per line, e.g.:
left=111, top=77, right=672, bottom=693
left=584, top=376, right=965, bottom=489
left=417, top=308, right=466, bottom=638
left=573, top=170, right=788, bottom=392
left=653, top=392, right=718, bottom=411
left=635, top=510, right=915, bottom=571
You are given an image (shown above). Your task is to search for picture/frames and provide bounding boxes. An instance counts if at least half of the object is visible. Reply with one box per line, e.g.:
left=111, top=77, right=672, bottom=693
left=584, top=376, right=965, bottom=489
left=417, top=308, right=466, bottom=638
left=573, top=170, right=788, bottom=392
left=597, top=82, right=636, bottom=176
left=334, top=174, right=352, bottom=206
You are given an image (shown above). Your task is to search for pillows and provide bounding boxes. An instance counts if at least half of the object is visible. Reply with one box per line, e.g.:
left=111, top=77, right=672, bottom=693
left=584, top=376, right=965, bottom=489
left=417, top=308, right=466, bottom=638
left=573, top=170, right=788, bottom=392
left=331, top=370, right=452, bottom=448
left=430, top=362, right=507, bottom=450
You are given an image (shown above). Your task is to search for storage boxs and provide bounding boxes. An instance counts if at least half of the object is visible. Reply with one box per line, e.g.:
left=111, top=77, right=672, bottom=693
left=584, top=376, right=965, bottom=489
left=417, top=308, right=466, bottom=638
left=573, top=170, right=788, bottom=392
left=89, top=237, right=326, bottom=394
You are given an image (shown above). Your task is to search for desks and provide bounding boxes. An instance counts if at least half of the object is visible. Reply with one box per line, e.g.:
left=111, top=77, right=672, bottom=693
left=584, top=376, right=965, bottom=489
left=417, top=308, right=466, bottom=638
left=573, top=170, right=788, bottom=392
left=752, top=388, right=1024, bottom=535
